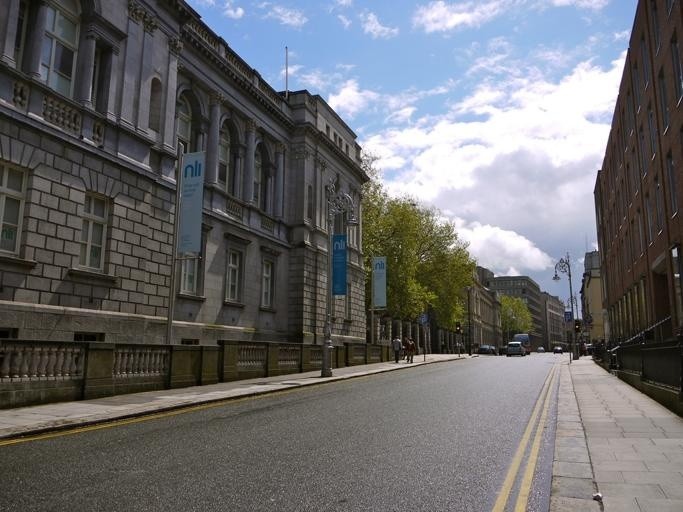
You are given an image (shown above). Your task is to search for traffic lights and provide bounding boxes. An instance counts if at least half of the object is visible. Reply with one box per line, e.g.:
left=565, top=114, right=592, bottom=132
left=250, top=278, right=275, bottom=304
left=456, top=322, right=460, bottom=331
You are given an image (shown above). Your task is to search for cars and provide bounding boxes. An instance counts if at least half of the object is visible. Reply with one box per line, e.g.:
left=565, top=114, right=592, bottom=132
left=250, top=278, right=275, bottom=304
left=537, top=347, right=544, bottom=353
left=480, top=342, right=526, bottom=357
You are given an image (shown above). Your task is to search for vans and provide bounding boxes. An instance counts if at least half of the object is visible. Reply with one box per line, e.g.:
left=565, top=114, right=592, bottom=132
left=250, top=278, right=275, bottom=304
left=512, top=334, right=532, bottom=355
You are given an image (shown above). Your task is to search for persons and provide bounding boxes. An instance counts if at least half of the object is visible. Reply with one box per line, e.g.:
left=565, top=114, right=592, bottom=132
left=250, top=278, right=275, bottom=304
left=407, top=337, right=416, bottom=363
left=392, top=335, right=402, bottom=363
left=402, top=337, right=410, bottom=360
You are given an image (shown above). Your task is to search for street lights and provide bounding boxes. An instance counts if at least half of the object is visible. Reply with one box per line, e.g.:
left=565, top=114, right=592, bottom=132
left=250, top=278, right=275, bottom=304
left=553, top=252, right=579, bottom=360
left=321, top=192, right=359, bottom=378
left=466, top=286, right=472, bottom=355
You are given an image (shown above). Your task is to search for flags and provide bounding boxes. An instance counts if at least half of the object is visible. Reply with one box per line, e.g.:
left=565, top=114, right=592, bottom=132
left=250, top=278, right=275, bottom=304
left=371, top=256, right=387, bottom=307
left=330, top=234, right=347, bottom=295
left=175, top=151, right=207, bottom=254
